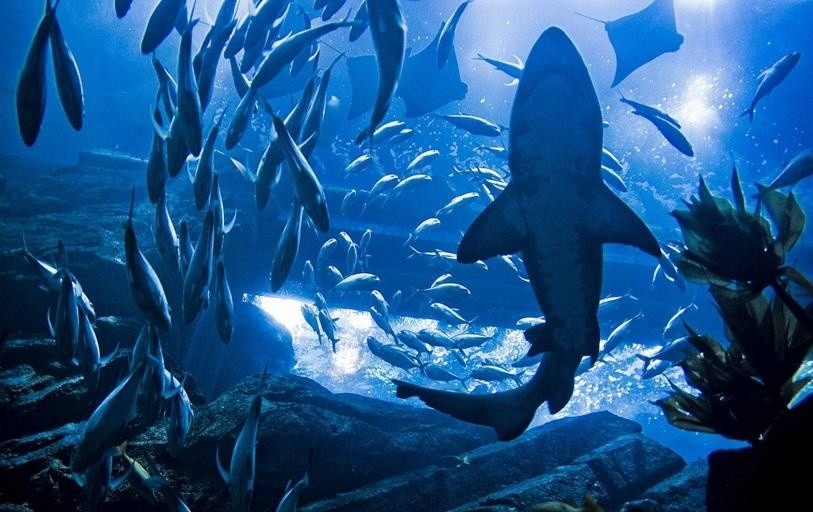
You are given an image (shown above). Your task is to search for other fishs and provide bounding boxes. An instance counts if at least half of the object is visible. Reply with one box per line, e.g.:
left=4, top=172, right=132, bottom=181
left=3, top=0, right=702, bottom=512
